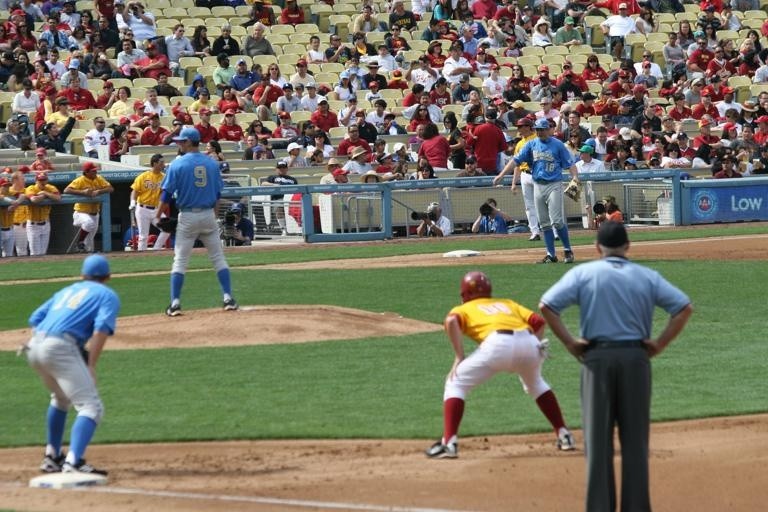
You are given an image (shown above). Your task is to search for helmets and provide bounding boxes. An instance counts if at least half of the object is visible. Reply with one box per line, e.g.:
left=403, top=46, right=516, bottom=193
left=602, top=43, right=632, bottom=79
left=460, top=270, right=492, bottom=300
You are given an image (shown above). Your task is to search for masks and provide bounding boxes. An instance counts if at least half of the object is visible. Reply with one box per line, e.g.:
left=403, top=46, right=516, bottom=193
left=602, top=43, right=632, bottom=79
left=231, top=202, right=247, bottom=213
left=287, top=142, right=302, bottom=152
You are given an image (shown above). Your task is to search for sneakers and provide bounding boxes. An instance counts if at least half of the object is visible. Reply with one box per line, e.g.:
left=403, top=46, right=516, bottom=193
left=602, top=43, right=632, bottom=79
left=40, top=452, right=66, bottom=472
left=564, top=250, right=574, bottom=263
left=224, top=297, right=239, bottom=311
left=78, top=240, right=85, bottom=250
left=166, top=304, right=181, bottom=316
left=424, top=435, right=457, bottom=458
left=558, top=434, right=575, bottom=450
left=536, top=255, right=559, bottom=263
left=61, top=458, right=108, bottom=476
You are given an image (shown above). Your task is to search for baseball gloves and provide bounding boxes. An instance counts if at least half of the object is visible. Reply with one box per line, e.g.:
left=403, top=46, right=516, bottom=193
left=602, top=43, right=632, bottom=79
left=564, top=179, right=581, bottom=202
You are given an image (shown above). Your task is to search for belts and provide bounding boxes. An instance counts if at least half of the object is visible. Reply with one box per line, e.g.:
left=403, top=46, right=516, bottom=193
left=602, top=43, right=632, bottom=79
left=497, top=330, right=514, bottom=335
left=89, top=212, right=97, bottom=215
left=1, top=228, right=10, bottom=231
left=135, top=202, right=154, bottom=209
left=521, top=169, right=532, bottom=174
left=30, top=221, right=45, bottom=225
left=583, top=340, right=643, bottom=350
left=14, top=222, right=26, bottom=226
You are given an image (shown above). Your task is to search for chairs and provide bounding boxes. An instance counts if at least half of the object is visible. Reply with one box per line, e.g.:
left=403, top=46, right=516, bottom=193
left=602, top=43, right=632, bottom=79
left=0, top=0, right=767, bottom=228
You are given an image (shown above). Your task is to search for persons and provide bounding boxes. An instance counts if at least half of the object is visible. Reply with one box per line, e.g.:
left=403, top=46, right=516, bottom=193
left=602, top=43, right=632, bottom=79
left=425, top=272, right=575, bottom=458
left=25, top=254, right=120, bottom=480
left=537, top=219, right=692, bottom=511
left=156, top=125, right=239, bottom=314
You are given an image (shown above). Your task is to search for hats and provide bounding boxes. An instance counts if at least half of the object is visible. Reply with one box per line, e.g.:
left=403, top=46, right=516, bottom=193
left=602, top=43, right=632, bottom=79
left=67, top=42, right=94, bottom=70
left=1, top=165, right=48, bottom=185
left=476, top=47, right=485, bottom=54
left=485, top=97, right=552, bottom=128
left=428, top=41, right=442, bottom=53
left=279, top=82, right=328, bottom=120
left=57, top=98, right=75, bottom=105
left=690, top=73, right=736, bottom=96
left=236, top=59, right=246, bottom=65
left=538, top=60, right=574, bottom=79
left=35, top=147, right=47, bottom=157
left=497, top=16, right=575, bottom=42
left=63, top=0, right=75, bottom=7
left=80, top=254, right=111, bottom=276
left=81, top=161, right=100, bottom=172
left=618, top=59, right=651, bottom=94
left=45, top=85, right=58, bottom=95
left=276, top=161, right=288, bottom=169
left=583, top=92, right=597, bottom=99
left=119, top=101, right=235, bottom=141
left=220, top=162, right=230, bottom=172
left=10, top=10, right=26, bottom=16
left=103, top=82, right=115, bottom=92
left=326, top=143, right=408, bottom=184
left=577, top=113, right=687, bottom=165
left=596, top=220, right=627, bottom=248
left=340, top=60, right=406, bottom=101
left=619, top=3, right=628, bottom=10
left=114, top=0, right=123, bottom=7
left=696, top=100, right=768, bottom=161
left=296, top=59, right=306, bottom=66
